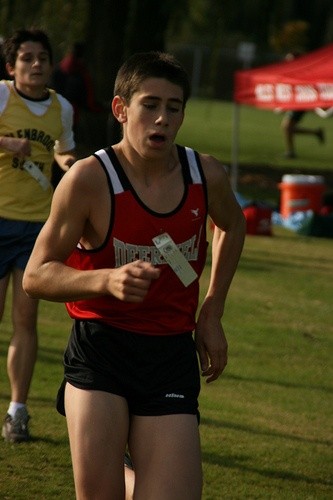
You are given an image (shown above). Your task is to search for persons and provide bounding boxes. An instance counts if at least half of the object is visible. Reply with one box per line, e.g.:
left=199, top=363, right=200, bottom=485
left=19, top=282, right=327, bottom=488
left=273, top=36, right=327, bottom=158
left=0, top=30, right=75, bottom=442
left=22, top=51, right=248, bottom=500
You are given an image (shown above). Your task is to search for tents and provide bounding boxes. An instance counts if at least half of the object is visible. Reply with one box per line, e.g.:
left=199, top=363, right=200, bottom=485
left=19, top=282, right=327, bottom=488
left=229, top=44, right=333, bottom=191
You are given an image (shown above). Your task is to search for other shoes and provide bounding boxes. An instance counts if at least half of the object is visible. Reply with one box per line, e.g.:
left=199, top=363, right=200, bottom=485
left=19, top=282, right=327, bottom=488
left=0, top=406, right=31, bottom=442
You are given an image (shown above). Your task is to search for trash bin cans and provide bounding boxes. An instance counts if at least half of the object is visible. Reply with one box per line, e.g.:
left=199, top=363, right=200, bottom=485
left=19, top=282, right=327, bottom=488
left=278, top=174, right=325, bottom=228
